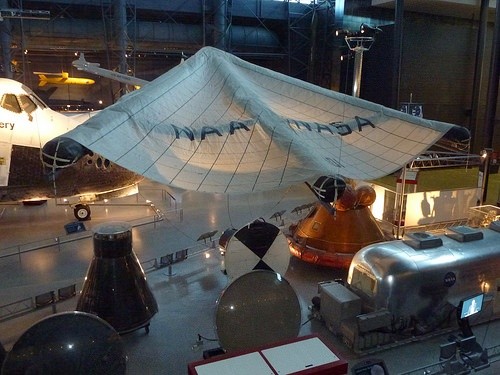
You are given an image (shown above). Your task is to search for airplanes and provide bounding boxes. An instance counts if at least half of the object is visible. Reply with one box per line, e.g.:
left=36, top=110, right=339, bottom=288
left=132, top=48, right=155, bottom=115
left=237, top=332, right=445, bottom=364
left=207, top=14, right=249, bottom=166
left=0, top=73, right=111, bottom=187
left=38, top=72, right=96, bottom=87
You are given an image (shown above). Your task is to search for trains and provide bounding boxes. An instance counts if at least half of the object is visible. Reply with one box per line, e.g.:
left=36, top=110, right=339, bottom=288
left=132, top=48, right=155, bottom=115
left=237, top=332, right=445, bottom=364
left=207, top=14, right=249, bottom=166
left=302, top=219, right=499, bottom=356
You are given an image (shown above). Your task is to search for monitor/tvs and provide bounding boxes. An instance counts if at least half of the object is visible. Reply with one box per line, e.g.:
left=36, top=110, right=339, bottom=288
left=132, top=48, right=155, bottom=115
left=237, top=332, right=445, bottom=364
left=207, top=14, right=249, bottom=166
left=458, top=293, right=485, bottom=320
